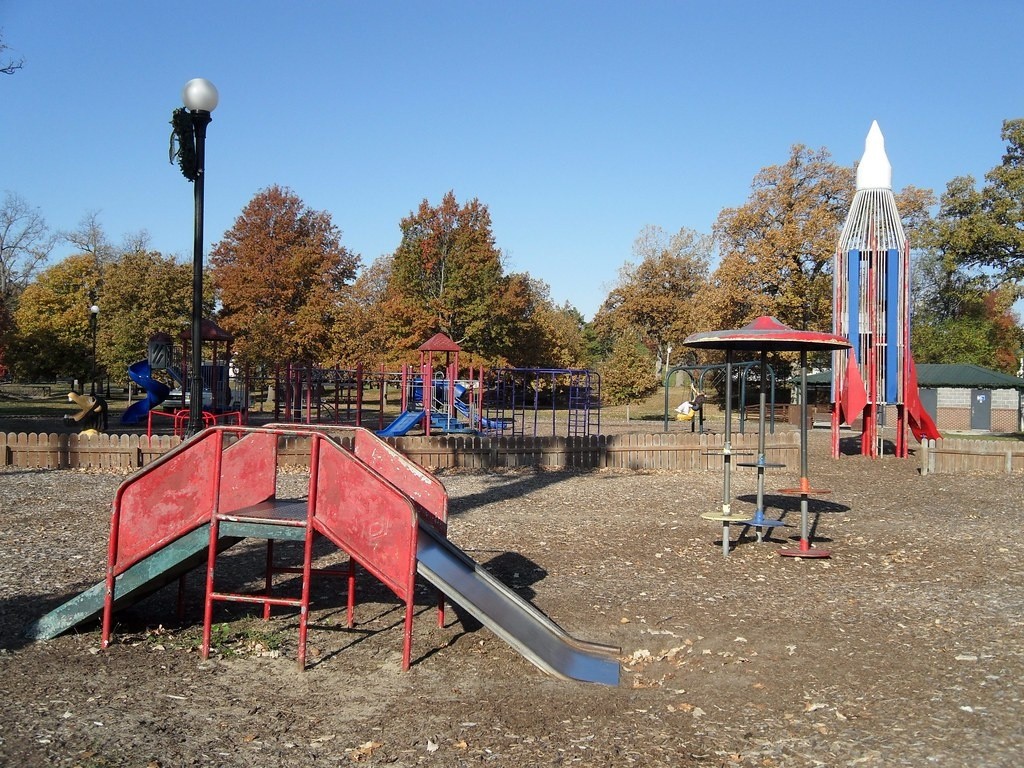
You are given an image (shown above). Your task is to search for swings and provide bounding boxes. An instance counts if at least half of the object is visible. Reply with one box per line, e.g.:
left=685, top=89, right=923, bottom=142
left=689, top=367, right=727, bottom=411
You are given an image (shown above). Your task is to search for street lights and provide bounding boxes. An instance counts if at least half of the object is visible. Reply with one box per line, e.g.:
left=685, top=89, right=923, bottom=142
left=90, top=305, right=99, bottom=395
left=164, top=78, right=218, bottom=438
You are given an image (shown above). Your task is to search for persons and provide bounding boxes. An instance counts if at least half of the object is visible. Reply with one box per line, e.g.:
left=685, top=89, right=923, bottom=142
left=90, top=393, right=108, bottom=431
left=675, top=383, right=707, bottom=414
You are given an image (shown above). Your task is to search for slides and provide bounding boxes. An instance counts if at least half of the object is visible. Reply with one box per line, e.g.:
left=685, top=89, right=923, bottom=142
left=416, top=516, right=623, bottom=687
left=453, top=397, right=507, bottom=428
left=375, top=410, right=426, bottom=436
left=120, top=358, right=170, bottom=425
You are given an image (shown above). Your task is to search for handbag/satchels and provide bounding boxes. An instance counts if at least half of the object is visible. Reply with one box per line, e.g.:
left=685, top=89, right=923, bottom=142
left=674, top=402, right=695, bottom=415
left=677, top=408, right=695, bottom=421
